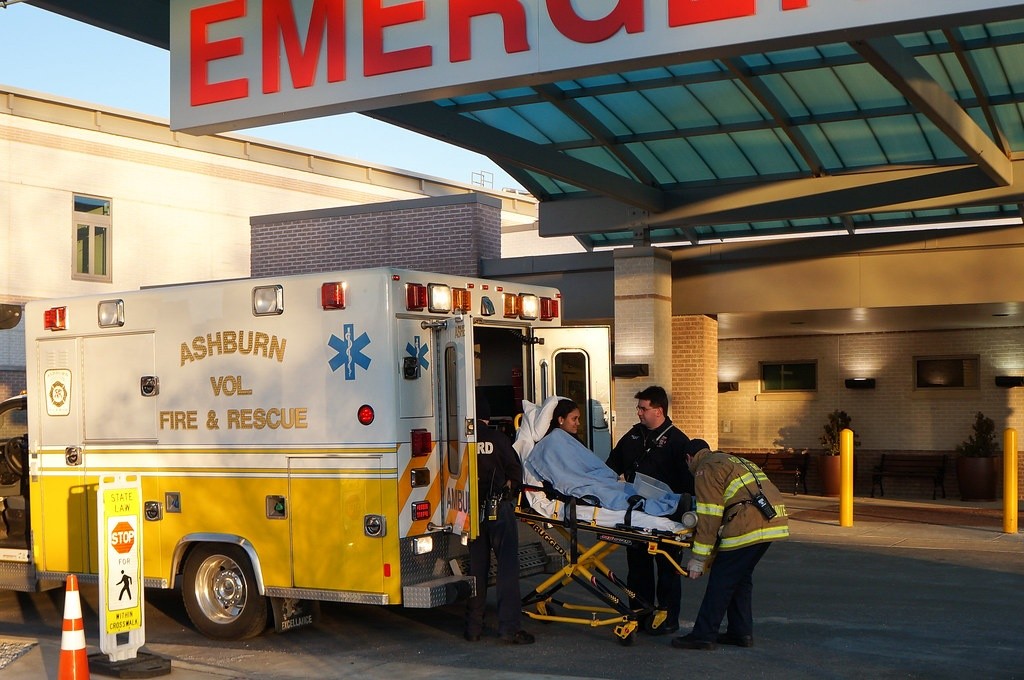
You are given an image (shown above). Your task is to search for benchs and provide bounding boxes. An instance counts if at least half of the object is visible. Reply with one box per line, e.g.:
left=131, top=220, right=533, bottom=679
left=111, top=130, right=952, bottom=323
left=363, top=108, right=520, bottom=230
left=870, top=453, right=949, bottom=500
left=760, top=452, right=809, bottom=497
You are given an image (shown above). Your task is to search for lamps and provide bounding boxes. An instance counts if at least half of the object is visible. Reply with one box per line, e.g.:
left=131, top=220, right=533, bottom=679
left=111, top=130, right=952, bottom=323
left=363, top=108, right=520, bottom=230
left=995, top=376, right=1024, bottom=388
left=718, top=382, right=738, bottom=393
left=844, top=377, right=876, bottom=389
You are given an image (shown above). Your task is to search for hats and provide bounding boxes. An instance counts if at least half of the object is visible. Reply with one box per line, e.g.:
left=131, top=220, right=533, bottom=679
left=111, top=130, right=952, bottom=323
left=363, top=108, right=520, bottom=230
left=476, top=393, right=490, bottom=417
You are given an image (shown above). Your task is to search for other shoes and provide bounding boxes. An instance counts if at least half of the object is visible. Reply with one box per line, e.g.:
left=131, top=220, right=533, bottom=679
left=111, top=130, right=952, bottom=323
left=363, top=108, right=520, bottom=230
left=671, top=632, right=716, bottom=650
left=716, top=632, right=754, bottom=647
left=664, top=619, right=679, bottom=632
left=463, top=630, right=480, bottom=642
left=499, top=628, right=535, bottom=644
left=669, top=492, right=693, bottom=524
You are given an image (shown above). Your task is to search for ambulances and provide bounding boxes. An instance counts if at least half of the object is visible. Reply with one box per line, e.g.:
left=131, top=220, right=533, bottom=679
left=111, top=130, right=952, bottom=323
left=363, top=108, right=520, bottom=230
left=0, top=267, right=618, bottom=643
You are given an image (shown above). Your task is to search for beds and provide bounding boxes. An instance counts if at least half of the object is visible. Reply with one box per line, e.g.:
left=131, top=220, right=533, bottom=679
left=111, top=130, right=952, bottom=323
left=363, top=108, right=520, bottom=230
left=510, top=415, right=711, bottom=647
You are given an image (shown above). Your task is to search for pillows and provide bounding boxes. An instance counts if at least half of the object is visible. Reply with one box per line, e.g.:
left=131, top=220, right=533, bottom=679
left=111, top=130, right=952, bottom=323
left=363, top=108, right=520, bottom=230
left=533, top=394, right=571, bottom=444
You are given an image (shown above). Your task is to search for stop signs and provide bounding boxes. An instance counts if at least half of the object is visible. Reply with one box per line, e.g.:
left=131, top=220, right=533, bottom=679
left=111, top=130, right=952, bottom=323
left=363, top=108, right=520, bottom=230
left=110, top=521, right=136, bottom=556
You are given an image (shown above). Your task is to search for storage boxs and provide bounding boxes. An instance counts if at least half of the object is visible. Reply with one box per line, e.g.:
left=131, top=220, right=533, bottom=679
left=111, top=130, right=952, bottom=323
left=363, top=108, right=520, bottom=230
left=632, top=471, right=674, bottom=502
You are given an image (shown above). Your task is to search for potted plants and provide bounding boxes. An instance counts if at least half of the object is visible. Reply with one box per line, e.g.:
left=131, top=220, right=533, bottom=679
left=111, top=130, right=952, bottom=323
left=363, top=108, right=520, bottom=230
left=954, top=410, right=1002, bottom=502
left=817, top=408, right=862, bottom=497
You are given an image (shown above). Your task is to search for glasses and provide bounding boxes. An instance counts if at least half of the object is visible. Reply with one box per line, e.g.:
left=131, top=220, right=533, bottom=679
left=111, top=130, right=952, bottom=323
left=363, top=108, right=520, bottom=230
left=636, top=406, right=656, bottom=412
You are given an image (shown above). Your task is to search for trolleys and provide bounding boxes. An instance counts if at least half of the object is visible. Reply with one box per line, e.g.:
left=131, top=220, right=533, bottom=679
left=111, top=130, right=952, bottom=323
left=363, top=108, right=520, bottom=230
left=511, top=481, right=703, bottom=647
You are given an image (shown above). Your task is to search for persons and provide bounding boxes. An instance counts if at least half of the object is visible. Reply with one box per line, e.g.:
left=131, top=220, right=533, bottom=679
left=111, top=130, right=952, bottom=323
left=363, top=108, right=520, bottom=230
left=465, top=408, right=537, bottom=645
left=672, top=438, right=789, bottom=648
left=604, top=386, right=691, bottom=635
left=527, top=400, right=694, bottom=524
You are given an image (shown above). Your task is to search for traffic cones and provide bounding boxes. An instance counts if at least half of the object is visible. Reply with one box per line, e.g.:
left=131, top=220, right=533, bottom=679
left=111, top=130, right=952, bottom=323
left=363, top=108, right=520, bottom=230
left=56, top=573, right=92, bottom=680
